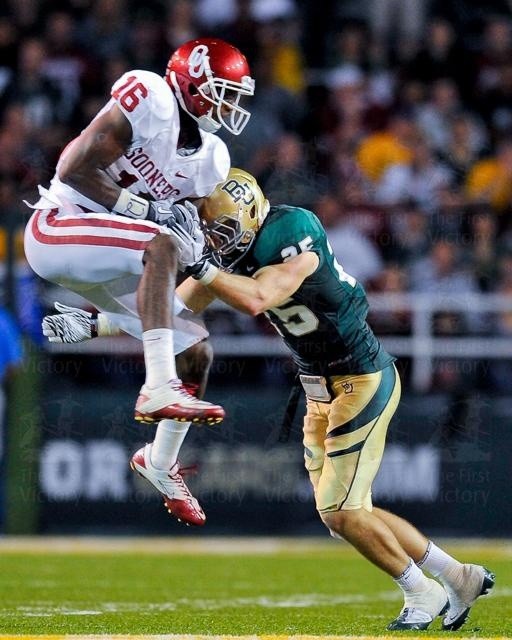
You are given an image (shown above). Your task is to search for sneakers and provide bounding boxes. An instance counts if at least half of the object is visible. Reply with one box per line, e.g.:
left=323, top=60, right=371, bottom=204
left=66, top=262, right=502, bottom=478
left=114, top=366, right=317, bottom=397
left=129, top=441, right=206, bottom=526
left=442, top=562, right=495, bottom=629
left=134, top=380, right=225, bottom=426
left=385, top=581, right=449, bottom=630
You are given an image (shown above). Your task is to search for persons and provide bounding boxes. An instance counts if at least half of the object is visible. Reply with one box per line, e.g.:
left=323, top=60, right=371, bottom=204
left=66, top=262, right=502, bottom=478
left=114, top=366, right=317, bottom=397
left=38, top=167, right=497, bottom=633
left=20, top=35, right=258, bottom=530
left=202, top=0, right=511, bottom=390
left=0, top=0, right=208, bottom=337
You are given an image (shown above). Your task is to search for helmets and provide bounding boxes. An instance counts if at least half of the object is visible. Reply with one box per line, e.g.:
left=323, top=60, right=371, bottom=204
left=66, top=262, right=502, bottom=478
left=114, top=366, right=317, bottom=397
left=200, top=168, right=270, bottom=245
left=166, top=37, right=251, bottom=118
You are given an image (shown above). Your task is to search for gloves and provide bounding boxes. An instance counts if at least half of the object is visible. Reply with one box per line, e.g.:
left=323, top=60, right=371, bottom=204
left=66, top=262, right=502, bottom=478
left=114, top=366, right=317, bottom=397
left=39, top=301, right=121, bottom=345
left=162, top=222, right=205, bottom=276
left=146, top=199, right=179, bottom=225
left=171, top=200, right=207, bottom=248
